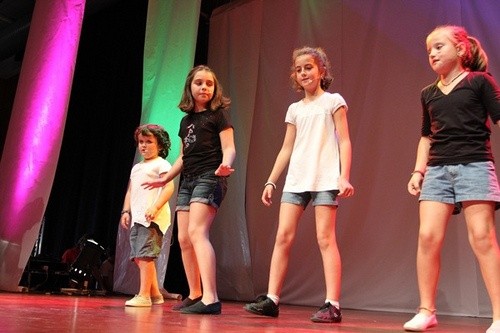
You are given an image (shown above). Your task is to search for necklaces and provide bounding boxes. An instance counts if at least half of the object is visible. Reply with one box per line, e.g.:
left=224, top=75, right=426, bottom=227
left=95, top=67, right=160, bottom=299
left=441, top=71, right=467, bottom=86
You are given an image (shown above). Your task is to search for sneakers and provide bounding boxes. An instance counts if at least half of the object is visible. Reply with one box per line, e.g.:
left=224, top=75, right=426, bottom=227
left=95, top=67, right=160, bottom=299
left=125, top=294, right=152, bottom=306
left=311, top=302, right=342, bottom=323
left=485, top=322, right=500, bottom=333
left=150, top=294, right=164, bottom=304
left=243, top=298, right=279, bottom=317
left=403, top=313, right=438, bottom=331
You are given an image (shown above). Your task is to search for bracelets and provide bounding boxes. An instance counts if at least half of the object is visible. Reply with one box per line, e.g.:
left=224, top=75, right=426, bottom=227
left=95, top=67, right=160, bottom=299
left=121, top=210, right=131, bottom=215
left=263, top=182, right=277, bottom=190
left=411, top=170, right=424, bottom=177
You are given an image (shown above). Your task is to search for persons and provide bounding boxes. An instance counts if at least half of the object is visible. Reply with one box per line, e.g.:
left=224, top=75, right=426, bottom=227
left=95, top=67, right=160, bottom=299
left=100, top=253, right=114, bottom=289
left=402, top=24, right=500, bottom=333
left=243, top=47, right=354, bottom=324
left=141, top=65, right=236, bottom=315
left=120, top=124, right=175, bottom=307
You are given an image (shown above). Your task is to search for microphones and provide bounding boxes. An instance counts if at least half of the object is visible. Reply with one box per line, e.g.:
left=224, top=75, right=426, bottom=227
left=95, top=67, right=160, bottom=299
left=437, top=51, right=442, bottom=53
left=145, top=150, right=148, bottom=152
left=309, top=77, right=319, bottom=82
left=209, top=92, right=212, bottom=94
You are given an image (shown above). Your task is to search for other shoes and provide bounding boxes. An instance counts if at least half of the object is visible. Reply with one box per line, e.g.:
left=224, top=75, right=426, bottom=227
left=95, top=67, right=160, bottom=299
left=171, top=295, right=203, bottom=309
left=174, top=300, right=221, bottom=315
